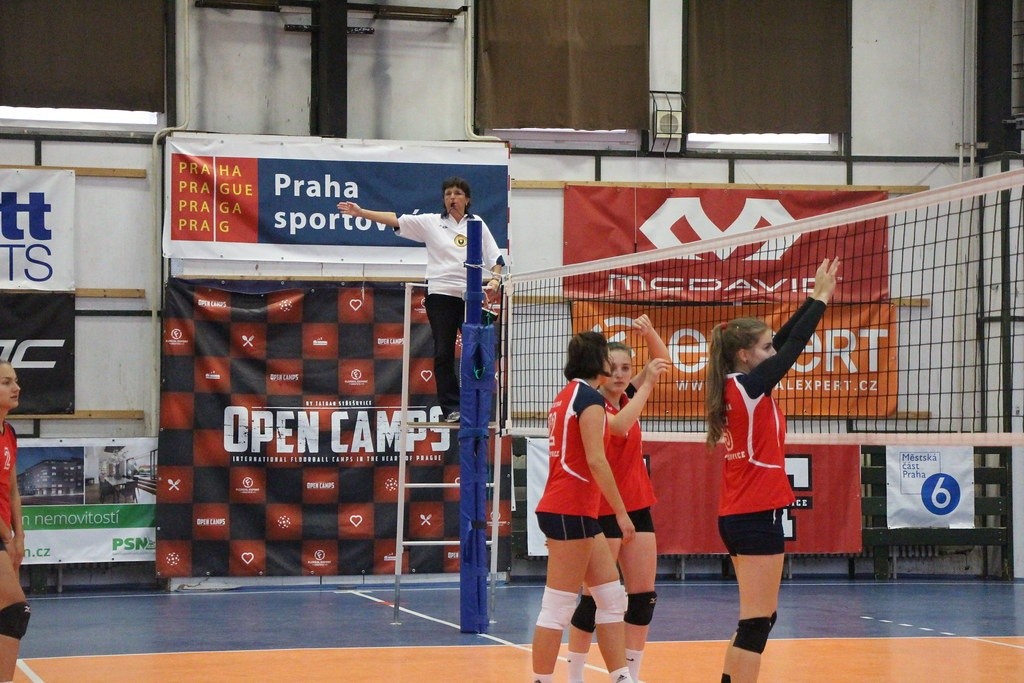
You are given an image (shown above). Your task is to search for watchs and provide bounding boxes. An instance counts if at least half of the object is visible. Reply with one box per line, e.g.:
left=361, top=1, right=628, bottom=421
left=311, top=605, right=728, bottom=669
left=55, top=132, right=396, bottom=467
left=490, top=276, right=501, bottom=283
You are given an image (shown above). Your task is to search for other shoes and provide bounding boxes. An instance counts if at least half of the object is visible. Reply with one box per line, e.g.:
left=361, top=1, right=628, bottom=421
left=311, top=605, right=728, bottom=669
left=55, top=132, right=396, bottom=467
left=445, top=411, right=460, bottom=423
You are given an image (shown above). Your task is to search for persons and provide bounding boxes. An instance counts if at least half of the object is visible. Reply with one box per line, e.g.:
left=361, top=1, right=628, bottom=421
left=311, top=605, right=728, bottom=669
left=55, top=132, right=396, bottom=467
left=0, top=357, right=33, bottom=683
left=704, top=253, right=842, bottom=683
left=529, top=314, right=676, bottom=683
left=336, top=176, right=505, bottom=424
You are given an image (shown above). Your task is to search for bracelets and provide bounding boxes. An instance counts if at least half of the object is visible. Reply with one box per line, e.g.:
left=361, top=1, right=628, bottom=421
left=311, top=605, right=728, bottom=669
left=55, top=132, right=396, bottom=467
left=15, top=534, right=25, bottom=539
left=3, top=538, right=13, bottom=542
